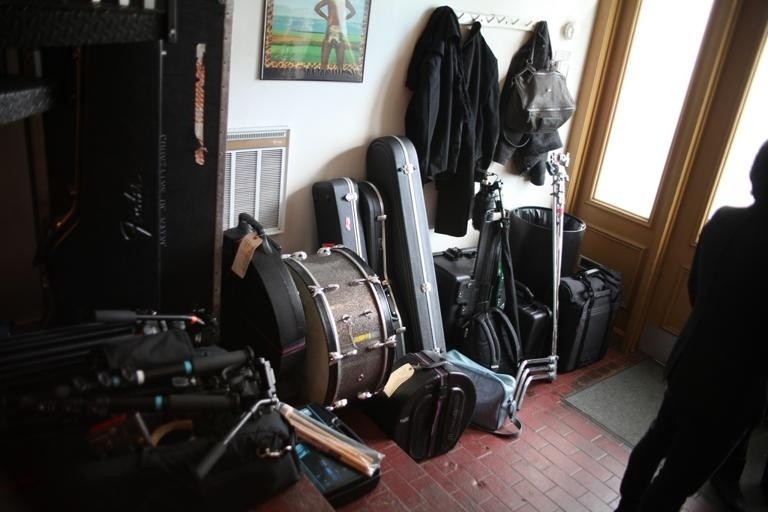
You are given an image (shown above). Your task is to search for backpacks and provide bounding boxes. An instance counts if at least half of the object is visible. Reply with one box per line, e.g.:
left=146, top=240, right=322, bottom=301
left=458, top=306, right=522, bottom=375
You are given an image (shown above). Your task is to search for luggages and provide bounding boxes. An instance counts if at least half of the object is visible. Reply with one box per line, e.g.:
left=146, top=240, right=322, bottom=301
left=550, top=264, right=621, bottom=374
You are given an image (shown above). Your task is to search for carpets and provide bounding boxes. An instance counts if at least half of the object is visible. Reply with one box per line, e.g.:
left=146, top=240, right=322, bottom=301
left=558, top=354, right=768, bottom=512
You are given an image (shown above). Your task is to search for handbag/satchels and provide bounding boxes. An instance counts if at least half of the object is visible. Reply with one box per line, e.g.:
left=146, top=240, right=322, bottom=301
left=441, top=350, right=522, bottom=438
left=500, top=23, right=575, bottom=136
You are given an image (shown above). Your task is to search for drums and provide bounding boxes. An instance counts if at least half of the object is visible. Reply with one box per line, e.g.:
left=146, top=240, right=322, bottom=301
left=284, top=246, right=397, bottom=411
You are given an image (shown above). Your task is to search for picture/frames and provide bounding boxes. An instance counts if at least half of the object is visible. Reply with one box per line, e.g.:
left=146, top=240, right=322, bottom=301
left=256, top=0, right=372, bottom=85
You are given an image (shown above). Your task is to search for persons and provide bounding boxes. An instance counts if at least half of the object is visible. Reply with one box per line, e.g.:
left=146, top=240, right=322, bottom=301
left=615, top=140, right=768, bottom=512
left=314, top=0, right=356, bottom=70
